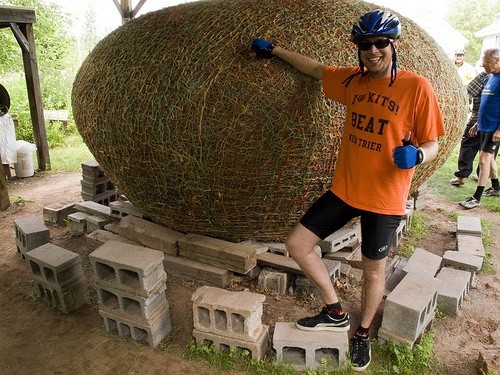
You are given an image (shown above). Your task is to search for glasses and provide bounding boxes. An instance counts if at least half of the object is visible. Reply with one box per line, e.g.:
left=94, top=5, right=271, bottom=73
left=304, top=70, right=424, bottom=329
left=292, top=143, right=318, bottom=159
left=358, top=39, right=394, bottom=50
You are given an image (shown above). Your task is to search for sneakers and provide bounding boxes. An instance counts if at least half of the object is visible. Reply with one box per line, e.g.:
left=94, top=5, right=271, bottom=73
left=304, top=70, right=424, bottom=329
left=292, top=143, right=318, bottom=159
left=295, top=307, right=350, bottom=333
left=459, top=196, right=480, bottom=209
left=483, top=189, right=500, bottom=196
left=350, top=337, right=371, bottom=372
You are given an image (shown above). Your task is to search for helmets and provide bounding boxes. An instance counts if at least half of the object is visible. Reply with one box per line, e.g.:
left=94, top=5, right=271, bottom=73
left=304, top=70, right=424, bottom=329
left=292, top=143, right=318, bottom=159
left=352, top=9, right=401, bottom=41
left=455, top=48, right=465, bottom=54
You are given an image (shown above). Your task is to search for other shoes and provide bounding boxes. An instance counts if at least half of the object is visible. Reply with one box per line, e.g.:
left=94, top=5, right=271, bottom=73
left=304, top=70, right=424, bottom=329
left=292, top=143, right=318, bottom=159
left=473, top=175, right=479, bottom=181
left=451, top=178, right=464, bottom=185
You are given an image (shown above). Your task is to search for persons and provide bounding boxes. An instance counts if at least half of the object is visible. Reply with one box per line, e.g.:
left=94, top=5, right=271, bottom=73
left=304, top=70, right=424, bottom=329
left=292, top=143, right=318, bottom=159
left=250, top=9, right=447, bottom=371
left=457, top=49, right=500, bottom=209
left=452, top=48, right=476, bottom=86
left=450, top=49, right=492, bottom=185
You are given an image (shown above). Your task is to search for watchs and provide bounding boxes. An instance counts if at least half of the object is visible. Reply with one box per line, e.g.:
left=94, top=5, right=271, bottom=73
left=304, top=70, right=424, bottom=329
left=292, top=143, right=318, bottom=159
left=417, top=148, right=424, bottom=164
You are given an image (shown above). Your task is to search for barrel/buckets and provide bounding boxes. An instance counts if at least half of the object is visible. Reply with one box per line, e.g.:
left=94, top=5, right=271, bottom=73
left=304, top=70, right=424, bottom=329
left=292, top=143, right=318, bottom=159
left=14, top=152, right=34, bottom=177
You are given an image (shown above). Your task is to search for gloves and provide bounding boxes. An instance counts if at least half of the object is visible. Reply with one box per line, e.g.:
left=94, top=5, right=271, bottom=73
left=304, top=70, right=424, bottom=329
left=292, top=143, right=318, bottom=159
left=251, top=39, right=275, bottom=59
left=393, top=145, right=417, bottom=169
